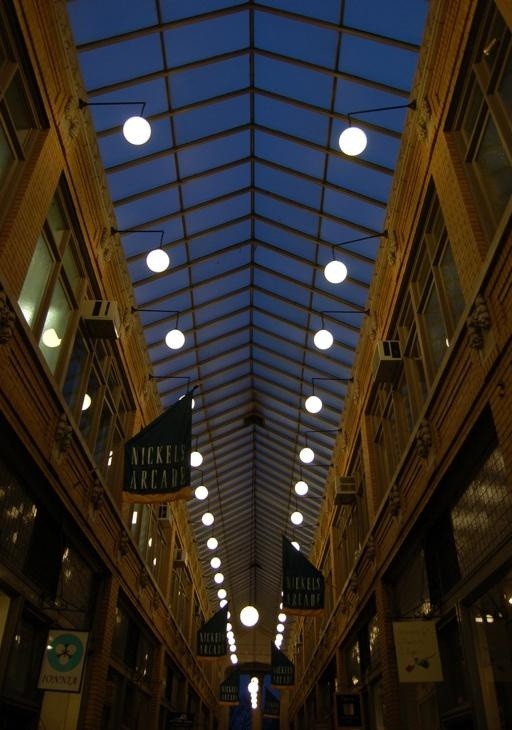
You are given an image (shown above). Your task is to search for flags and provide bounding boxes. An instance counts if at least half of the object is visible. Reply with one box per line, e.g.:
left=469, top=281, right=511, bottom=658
left=263, top=686, right=280, bottom=715
left=282, top=535, right=325, bottom=617
left=219, top=666, right=239, bottom=702
left=271, top=639, right=295, bottom=685
left=197, top=603, right=227, bottom=657
left=122, top=389, right=190, bottom=504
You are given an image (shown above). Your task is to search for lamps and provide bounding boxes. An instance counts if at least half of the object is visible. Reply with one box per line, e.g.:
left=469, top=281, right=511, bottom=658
left=314, top=309, right=370, bottom=350
left=79, top=98, right=151, bottom=146
left=111, top=226, right=170, bottom=274
left=339, top=100, right=416, bottom=156
left=324, top=230, right=388, bottom=284
left=131, top=306, right=185, bottom=350
